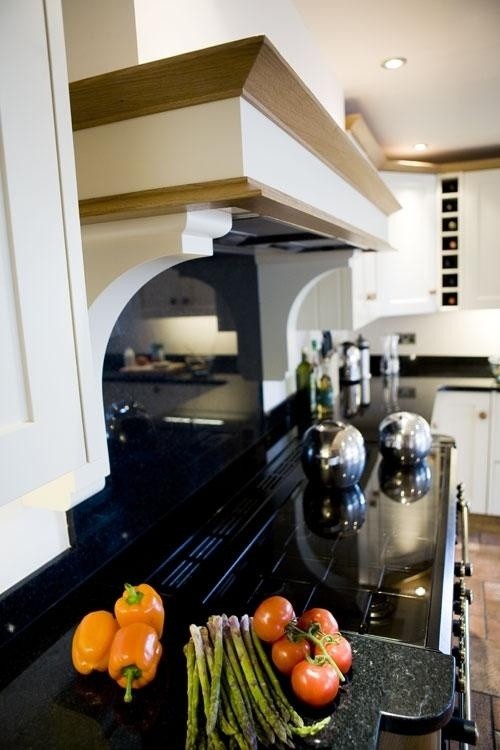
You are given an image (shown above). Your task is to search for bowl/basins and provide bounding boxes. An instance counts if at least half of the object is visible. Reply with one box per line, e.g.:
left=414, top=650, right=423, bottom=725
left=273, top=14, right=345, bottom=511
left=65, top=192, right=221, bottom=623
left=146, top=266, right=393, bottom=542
left=184, top=355, right=217, bottom=377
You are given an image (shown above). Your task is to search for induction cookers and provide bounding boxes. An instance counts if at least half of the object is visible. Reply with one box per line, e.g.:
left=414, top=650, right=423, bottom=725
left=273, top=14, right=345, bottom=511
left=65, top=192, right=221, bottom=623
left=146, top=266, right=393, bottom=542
left=143, top=423, right=453, bottom=652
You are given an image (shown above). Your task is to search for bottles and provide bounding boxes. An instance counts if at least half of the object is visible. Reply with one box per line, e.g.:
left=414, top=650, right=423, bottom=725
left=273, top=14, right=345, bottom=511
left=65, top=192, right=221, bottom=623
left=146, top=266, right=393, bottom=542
left=362, top=380, right=372, bottom=408
left=342, top=383, right=361, bottom=419
left=316, top=398, right=334, bottom=421
left=123, top=344, right=134, bottom=367
left=150, top=343, right=165, bottom=362
left=296, top=330, right=372, bottom=398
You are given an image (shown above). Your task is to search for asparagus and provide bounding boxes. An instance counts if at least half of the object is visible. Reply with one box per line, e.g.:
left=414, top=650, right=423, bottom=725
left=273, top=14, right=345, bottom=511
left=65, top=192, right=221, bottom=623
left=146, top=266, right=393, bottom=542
left=181, top=613, right=330, bottom=750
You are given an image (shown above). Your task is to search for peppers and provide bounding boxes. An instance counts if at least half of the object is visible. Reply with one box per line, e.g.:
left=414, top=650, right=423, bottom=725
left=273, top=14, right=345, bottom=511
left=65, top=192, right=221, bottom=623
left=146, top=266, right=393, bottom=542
left=70, top=580, right=165, bottom=702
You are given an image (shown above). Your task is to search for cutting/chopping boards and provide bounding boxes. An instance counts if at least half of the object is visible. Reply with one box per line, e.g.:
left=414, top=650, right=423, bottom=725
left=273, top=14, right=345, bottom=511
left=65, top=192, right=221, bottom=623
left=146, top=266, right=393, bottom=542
left=119, top=360, right=186, bottom=378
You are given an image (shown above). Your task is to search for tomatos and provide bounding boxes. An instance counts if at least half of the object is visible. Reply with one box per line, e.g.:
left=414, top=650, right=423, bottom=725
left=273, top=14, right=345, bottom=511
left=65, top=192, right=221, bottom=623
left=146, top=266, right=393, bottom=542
left=253, top=596, right=353, bottom=706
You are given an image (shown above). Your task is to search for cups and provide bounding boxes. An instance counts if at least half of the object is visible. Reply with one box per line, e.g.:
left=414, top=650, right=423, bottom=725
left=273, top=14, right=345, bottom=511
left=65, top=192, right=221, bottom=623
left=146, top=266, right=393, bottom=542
left=381, top=375, right=400, bottom=414
left=379, top=335, right=400, bottom=374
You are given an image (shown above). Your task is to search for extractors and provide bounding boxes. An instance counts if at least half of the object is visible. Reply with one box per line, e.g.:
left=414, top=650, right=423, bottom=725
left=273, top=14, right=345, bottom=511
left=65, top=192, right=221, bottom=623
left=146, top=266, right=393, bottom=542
left=214, top=211, right=360, bottom=255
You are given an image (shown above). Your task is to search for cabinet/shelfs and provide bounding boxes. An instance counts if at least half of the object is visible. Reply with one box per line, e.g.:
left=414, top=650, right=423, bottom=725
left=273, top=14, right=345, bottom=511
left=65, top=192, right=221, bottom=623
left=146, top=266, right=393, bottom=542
left=139, top=281, right=218, bottom=321
left=428, top=386, right=499, bottom=517
left=295, top=161, right=500, bottom=332
